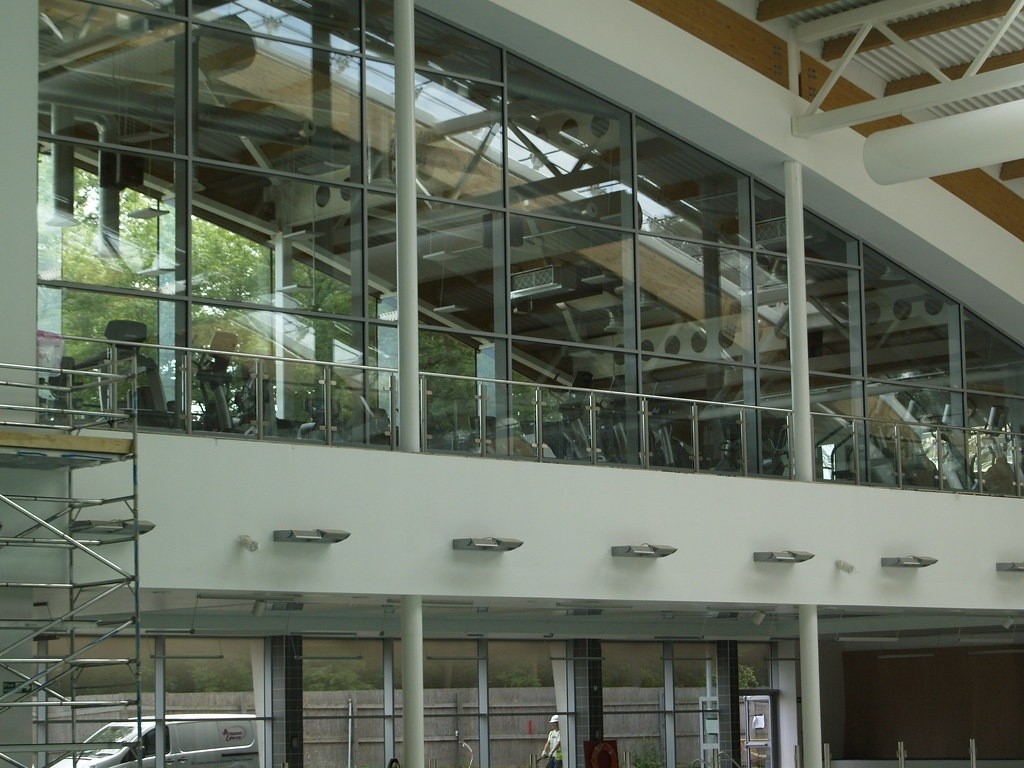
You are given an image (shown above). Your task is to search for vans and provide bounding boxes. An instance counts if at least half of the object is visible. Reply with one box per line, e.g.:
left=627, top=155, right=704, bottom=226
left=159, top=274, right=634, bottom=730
left=50, top=713, right=258, bottom=768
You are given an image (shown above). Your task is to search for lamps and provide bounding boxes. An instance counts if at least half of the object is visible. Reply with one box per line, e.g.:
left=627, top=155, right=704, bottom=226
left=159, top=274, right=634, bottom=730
left=449, top=539, right=524, bottom=551
left=754, top=552, right=816, bottom=562
left=882, top=556, right=939, bottom=567
left=271, top=529, right=351, bottom=543
left=611, top=547, right=679, bottom=557
left=69, top=519, right=156, bottom=535
left=996, top=562, right=1024, bottom=571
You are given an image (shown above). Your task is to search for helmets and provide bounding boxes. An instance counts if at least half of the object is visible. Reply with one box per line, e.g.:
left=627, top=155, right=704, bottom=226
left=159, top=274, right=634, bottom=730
left=550, top=715, right=558, bottom=722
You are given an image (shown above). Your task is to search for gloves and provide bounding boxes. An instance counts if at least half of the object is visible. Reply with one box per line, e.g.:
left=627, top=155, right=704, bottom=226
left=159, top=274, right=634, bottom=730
left=542, top=751, right=547, bottom=758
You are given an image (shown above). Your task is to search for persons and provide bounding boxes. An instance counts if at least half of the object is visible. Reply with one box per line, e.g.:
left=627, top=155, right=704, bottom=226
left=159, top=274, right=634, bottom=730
left=387, top=758, right=401, bottom=768
left=540, top=715, right=563, bottom=768
